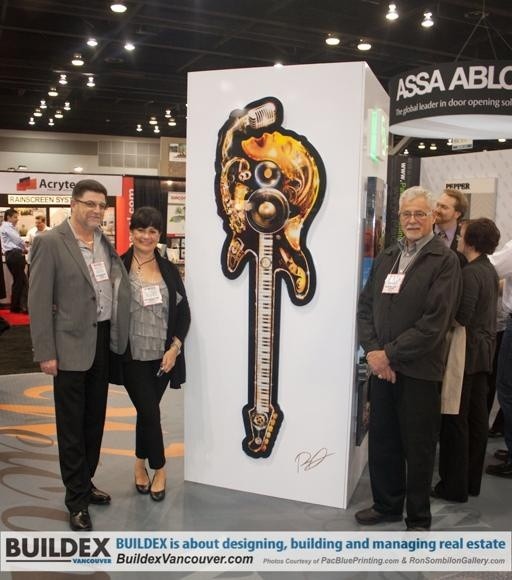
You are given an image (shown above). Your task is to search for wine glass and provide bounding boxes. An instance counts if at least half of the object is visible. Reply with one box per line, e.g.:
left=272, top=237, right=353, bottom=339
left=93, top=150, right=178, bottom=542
left=25, top=238, right=29, bottom=249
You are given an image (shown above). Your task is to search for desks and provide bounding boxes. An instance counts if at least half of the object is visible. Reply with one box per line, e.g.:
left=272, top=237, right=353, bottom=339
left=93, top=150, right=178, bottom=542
left=0, top=253, right=28, bottom=305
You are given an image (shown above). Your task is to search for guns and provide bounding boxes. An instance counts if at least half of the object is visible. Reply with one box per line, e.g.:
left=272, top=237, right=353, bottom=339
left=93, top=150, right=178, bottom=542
left=215, top=96, right=326, bottom=459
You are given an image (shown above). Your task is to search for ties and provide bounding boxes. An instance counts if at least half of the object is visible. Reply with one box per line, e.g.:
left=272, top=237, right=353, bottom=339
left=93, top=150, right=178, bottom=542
left=438, top=231, right=447, bottom=241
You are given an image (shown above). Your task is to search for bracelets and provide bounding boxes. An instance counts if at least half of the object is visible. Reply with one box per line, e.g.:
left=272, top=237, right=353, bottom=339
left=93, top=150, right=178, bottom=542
left=170, top=343, right=181, bottom=356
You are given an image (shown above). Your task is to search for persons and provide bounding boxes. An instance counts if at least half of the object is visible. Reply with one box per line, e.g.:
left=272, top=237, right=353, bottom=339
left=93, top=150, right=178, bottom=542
left=485, top=240, right=512, bottom=479
left=433, top=187, right=468, bottom=268
left=0, top=209, right=29, bottom=314
left=52, top=206, right=191, bottom=503
left=354, top=186, right=463, bottom=531
left=430, top=217, right=500, bottom=502
left=27, top=179, right=131, bottom=531
left=25, top=216, right=53, bottom=279
left=486, top=280, right=505, bottom=437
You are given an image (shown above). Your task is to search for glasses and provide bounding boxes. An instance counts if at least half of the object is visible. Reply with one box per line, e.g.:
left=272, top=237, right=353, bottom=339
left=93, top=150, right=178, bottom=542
left=399, top=211, right=433, bottom=219
left=73, top=198, right=106, bottom=212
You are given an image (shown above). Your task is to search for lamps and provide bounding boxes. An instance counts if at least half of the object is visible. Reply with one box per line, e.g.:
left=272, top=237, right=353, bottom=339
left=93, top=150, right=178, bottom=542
left=27, top=0, right=188, bottom=134
left=323, top=0, right=436, bottom=53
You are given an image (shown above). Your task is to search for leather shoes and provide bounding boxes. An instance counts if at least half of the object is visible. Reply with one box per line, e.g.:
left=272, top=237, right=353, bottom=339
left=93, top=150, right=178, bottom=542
left=134, top=467, right=151, bottom=494
left=88, top=481, right=111, bottom=504
left=494, top=449, right=511, bottom=461
left=69, top=503, right=92, bottom=532
left=9, top=304, right=29, bottom=315
left=429, top=485, right=468, bottom=502
left=149, top=472, right=167, bottom=501
left=485, top=462, right=512, bottom=479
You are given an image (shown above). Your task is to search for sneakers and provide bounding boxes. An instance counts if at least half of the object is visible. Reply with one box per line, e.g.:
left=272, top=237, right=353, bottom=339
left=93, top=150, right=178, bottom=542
left=354, top=506, right=403, bottom=526
left=406, top=523, right=431, bottom=532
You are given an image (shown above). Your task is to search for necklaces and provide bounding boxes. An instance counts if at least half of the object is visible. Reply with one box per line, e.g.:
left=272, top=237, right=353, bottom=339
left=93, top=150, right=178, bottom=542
left=81, top=238, right=93, bottom=244
left=134, top=255, right=156, bottom=274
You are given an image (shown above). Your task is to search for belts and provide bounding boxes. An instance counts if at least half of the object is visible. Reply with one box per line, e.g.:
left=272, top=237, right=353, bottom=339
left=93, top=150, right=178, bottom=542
left=5, top=249, right=14, bottom=255
